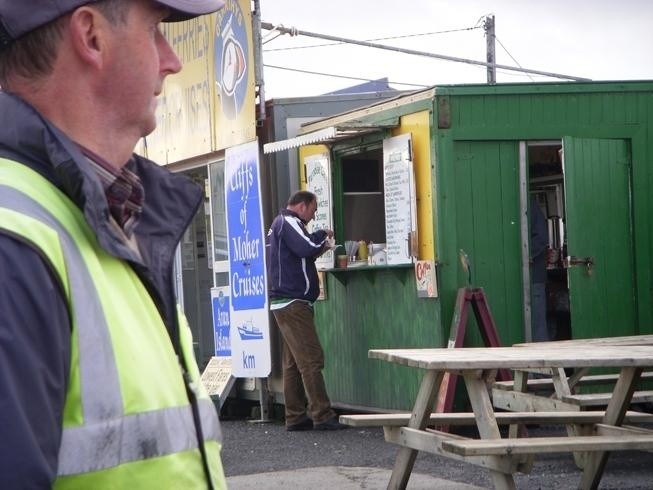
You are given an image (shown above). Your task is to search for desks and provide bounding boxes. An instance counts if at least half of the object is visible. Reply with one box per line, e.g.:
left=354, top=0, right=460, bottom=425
left=369, top=335, right=653, bottom=489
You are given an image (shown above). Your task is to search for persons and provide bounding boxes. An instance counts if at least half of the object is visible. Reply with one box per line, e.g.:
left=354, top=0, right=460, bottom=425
left=265, top=191, right=353, bottom=431
left=0, top=2, right=232, bottom=490
left=528, top=193, right=549, bottom=343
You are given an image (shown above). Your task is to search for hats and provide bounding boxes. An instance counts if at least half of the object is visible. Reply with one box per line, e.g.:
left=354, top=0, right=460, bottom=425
left=1, top=0, right=226, bottom=37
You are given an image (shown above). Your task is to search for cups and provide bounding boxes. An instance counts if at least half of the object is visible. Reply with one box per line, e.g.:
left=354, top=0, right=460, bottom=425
left=338, top=255, right=347, bottom=268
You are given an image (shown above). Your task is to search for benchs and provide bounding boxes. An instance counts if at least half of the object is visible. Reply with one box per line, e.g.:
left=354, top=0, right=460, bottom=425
left=339, top=372, right=651, bottom=457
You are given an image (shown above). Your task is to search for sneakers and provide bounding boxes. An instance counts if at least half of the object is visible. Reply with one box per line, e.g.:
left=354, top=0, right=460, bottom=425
left=286, top=417, right=348, bottom=431
